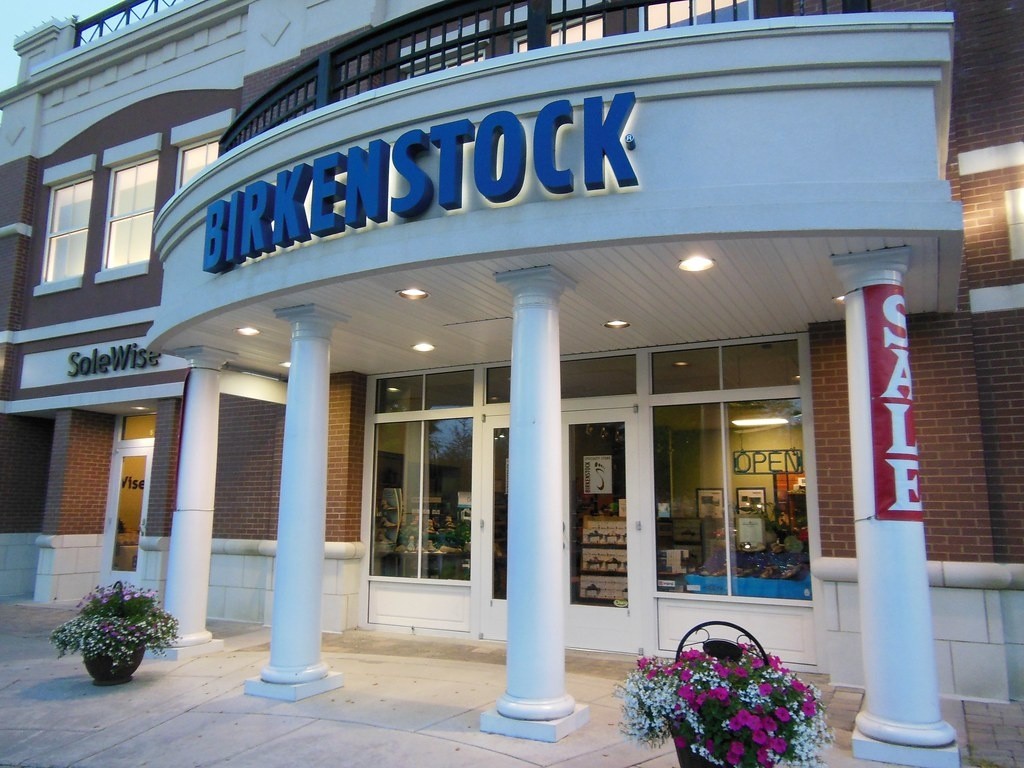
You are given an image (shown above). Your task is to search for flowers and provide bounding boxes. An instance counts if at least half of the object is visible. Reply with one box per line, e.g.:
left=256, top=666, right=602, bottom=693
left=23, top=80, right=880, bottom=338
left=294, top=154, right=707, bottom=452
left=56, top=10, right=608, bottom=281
left=48, top=583, right=181, bottom=673
left=610, top=646, right=835, bottom=768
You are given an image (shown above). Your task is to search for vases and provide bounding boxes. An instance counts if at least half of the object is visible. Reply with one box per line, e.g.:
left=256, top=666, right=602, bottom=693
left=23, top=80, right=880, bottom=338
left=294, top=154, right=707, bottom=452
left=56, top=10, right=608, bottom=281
left=83, top=647, right=145, bottom=686
left=672, top=732, right=729, bottom=768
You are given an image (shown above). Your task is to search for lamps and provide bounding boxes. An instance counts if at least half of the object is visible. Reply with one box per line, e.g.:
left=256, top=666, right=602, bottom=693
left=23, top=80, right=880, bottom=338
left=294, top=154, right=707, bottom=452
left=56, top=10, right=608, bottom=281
left=731, top=404, right=788, bottom=425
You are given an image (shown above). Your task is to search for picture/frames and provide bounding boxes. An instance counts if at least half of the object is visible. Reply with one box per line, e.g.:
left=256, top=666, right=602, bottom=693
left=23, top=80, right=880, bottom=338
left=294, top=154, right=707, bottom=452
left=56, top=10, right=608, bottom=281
left=695, top=487, right=725, bottom=518
left=736, top=486, right=766, bottom=515
